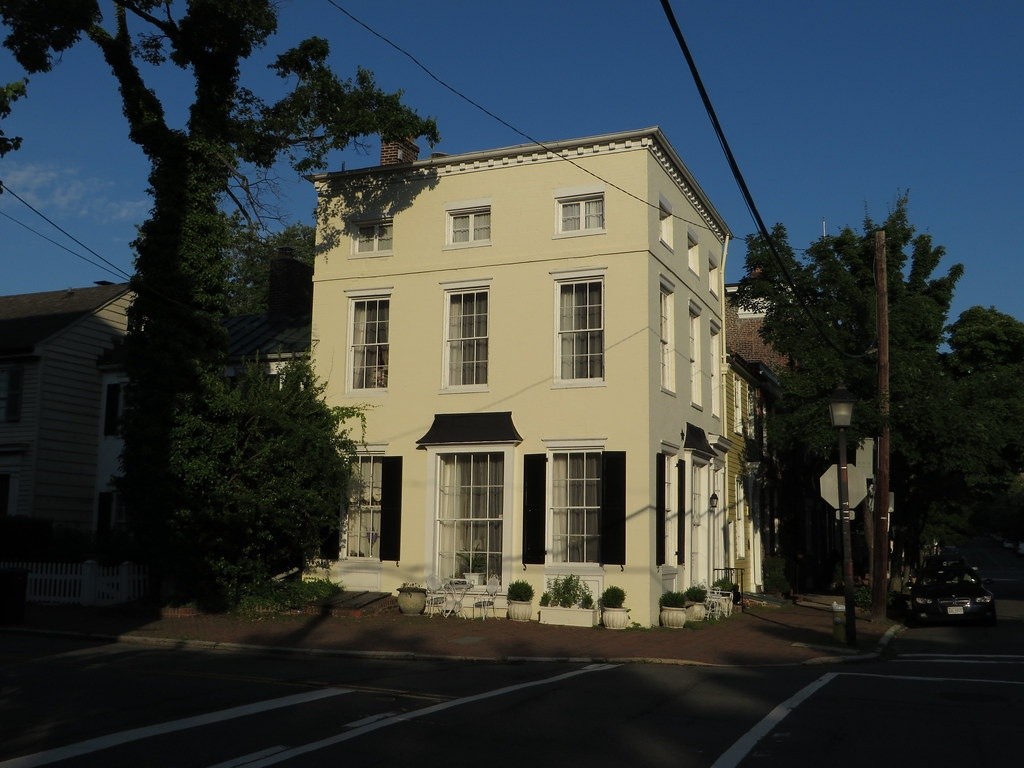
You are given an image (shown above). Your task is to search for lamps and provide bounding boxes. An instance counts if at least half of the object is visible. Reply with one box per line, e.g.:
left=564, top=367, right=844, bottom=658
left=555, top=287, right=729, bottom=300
left=709, top=491, right=718, bottom=512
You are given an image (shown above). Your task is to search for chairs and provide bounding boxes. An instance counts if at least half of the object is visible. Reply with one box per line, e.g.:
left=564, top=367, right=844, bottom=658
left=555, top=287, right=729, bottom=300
left=472, top=575, right=502, bottom=621
left=425, top=574, right=448, bottom=618
left=693, top=578, right=718, bottom=621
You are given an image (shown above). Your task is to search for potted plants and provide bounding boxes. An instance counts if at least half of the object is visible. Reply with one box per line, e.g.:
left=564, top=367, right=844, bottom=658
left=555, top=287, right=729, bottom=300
left=456, top=542, right=493, bottom=585
left=733, top=583, right=742, bottom=614
left=601, top=584, right=628, bottom=630
left=659, top=590, right=686, bottom=629
left=396, top=582, right=426, bottom=616
left=507, top=580, right=536, bottom=622
left=685, top=587, right=708, bottom=621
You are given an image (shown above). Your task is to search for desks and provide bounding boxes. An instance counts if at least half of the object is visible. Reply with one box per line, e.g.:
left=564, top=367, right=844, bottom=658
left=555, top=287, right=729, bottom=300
left=441, top=583, right=474, bottom=619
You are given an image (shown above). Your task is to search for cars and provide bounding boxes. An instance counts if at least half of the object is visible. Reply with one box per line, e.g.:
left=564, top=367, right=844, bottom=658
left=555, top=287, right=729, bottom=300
left=905, top=546, right=997, bottom=627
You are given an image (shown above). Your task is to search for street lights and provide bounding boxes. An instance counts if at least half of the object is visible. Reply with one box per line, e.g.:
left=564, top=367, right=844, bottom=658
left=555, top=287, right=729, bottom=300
left=827, top=376, right=858, bottom=646
